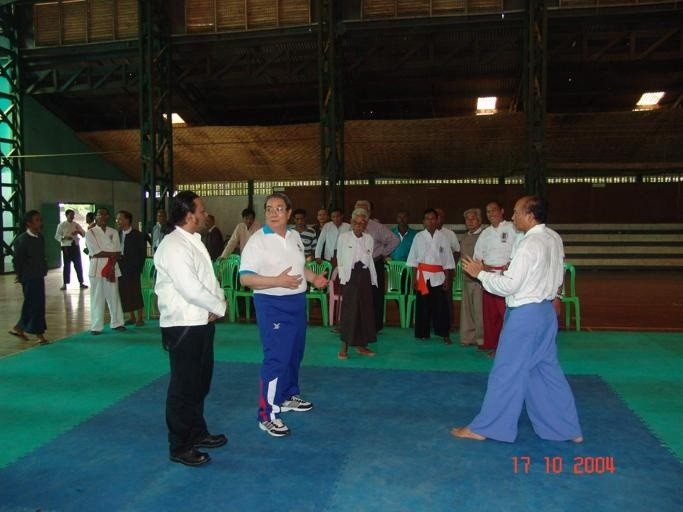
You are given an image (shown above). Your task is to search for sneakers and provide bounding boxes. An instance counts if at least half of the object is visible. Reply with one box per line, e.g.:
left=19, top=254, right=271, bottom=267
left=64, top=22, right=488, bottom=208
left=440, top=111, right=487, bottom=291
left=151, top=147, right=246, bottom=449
left=91, top=321, right=147, bottom=336
left=258, top=418, right=290, bottom=438
left=420, top=335, right=497, bottom=359
left=280, top=395, right=314, bottom=412
left=338, top=351, right=350, bottom=359
left=358, top=348, right=377, bottom=356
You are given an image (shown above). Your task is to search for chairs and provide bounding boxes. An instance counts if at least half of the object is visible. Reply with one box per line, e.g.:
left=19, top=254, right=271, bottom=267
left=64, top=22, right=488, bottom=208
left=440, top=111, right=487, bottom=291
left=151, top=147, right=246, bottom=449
left=306, top=259, right=344, bottom=328
left=214, top=253, right=255, bottom=323
left=557, top=264, right=580, bottom=330
left=140, top=257, right=157, bottom=323
left=381, top=260, right=419, bottom=331
left=453, top=255, right=464, bottom=302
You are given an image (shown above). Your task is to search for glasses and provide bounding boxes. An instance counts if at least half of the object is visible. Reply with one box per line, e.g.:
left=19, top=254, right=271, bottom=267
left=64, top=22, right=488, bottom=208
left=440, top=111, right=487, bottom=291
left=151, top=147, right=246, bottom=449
left=264, top=206, right=288, bottom=214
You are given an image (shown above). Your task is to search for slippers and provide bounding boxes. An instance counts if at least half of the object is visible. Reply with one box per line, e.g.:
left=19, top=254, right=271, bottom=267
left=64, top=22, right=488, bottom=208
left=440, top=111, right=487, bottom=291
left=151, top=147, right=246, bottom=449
left=37, top=336, right=49, bottom=345
left=7, top=330, right=30, bottom=342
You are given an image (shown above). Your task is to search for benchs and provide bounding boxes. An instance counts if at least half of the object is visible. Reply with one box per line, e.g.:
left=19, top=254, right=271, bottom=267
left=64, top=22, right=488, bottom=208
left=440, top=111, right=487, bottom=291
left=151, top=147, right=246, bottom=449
left=408, top=223, right=424, bottom=233
left=480, top=223, right=489, bottom=229
left=443, top=223, right=468, bottom=242
left=538, top=221, right=682, bottom=271
left=382, top=225, right=396, bottom=230
left=288, top=224, right=313, bottom=229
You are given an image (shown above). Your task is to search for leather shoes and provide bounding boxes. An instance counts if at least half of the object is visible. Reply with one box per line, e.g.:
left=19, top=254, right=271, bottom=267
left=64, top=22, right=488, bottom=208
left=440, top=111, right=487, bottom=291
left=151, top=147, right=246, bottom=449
left=168, top=447, right=212, bottom=466
left=191, top=431, right=229, bottom=449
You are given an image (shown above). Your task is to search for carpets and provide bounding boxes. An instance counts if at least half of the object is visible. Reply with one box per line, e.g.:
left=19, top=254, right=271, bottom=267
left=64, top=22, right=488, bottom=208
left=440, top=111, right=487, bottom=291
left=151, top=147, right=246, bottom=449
left=0, top=312, right=683, bottom=512
